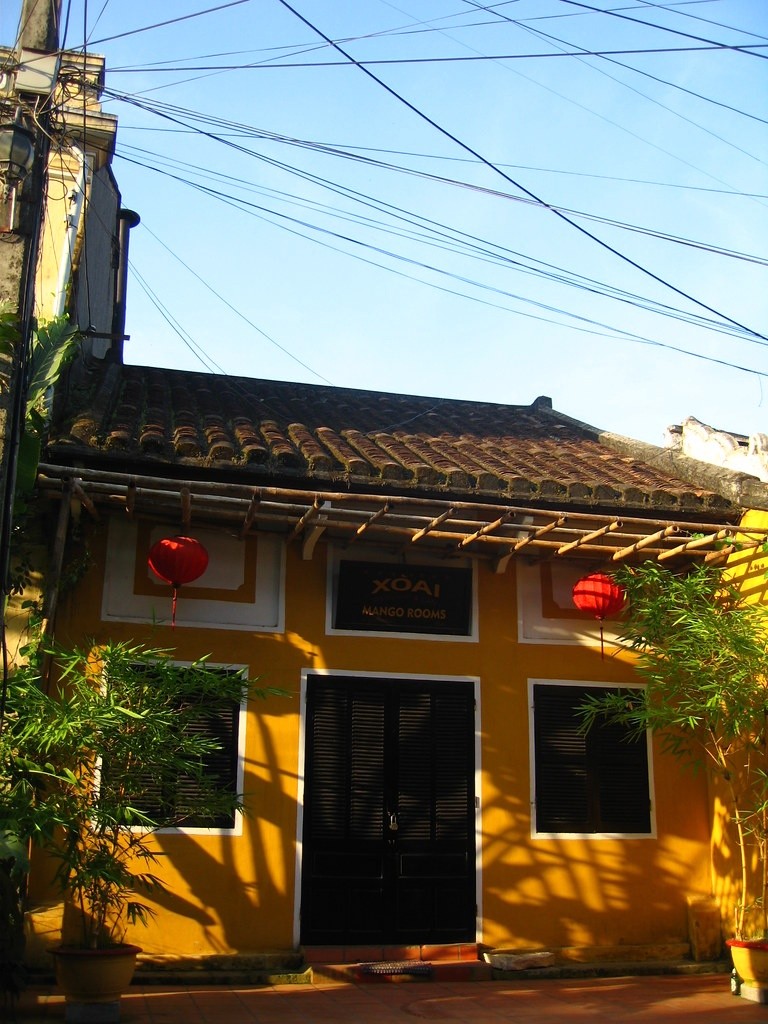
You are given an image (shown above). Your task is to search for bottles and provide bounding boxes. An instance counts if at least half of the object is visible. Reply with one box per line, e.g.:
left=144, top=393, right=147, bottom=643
left=730, top=968, right=740, bottom=995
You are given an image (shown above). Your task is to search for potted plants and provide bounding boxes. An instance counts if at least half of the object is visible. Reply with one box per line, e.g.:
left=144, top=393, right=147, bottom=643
left=572, top=530, right=768, bottom=986
left=0, top=637, right=299, bottom=1009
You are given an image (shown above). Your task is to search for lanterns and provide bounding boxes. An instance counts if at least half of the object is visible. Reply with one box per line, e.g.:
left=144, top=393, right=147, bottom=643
left=144, top=519, right=210, bottom=630
left=573, top=571, right=634, bottom=662
